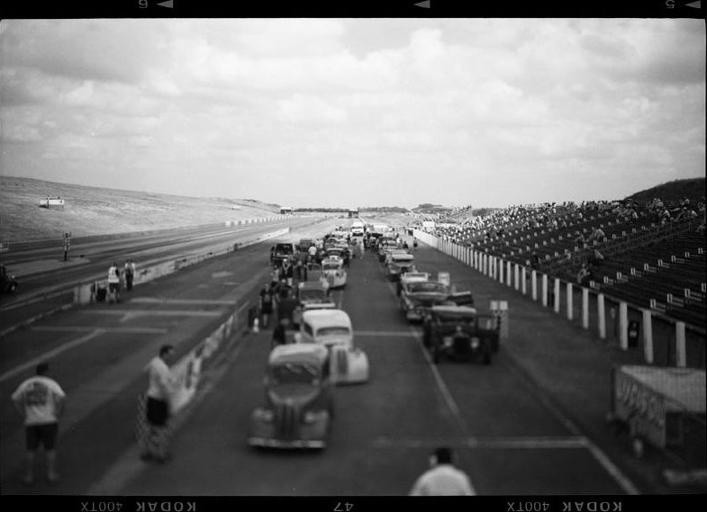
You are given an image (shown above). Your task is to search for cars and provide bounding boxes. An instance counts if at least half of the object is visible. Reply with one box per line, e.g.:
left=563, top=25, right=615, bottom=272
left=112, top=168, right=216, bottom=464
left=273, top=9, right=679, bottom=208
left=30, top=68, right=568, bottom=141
left=0, top=264, right=18, bottom=295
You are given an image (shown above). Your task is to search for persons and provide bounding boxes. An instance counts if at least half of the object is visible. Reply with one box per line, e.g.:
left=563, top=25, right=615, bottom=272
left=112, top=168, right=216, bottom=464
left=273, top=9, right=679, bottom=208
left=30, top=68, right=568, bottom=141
left=12, top=362, right=66, bottom=486
left=108, top=262, right=120, bottom=303
left=141, top=345, right=173, bottom=463
left=409, top=447, right=476, bottom=496
left=443, top=198, right=698, bottom=283
left=258, top=239, right=324, bottom=346
left=63, top=231, right=71, bottom=260
left=125, top=258, right=135, bottom=291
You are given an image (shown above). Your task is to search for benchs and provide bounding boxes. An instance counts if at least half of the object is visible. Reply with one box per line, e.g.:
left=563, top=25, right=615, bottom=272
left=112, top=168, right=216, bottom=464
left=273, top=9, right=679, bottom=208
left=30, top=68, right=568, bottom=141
left=448, top=209, right=707, bottom=329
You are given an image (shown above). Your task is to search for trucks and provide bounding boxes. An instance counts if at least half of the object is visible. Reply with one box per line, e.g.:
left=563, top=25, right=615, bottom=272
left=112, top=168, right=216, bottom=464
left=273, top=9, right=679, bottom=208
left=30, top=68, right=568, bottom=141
left=606, top=360, right=707, bottom=488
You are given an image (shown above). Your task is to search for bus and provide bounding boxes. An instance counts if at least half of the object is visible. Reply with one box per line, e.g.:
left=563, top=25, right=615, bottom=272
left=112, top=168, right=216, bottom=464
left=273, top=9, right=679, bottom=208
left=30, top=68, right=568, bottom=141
left=348, top=208, right=358, bottom=219
left=279, top=206, right=294, bottom=215
